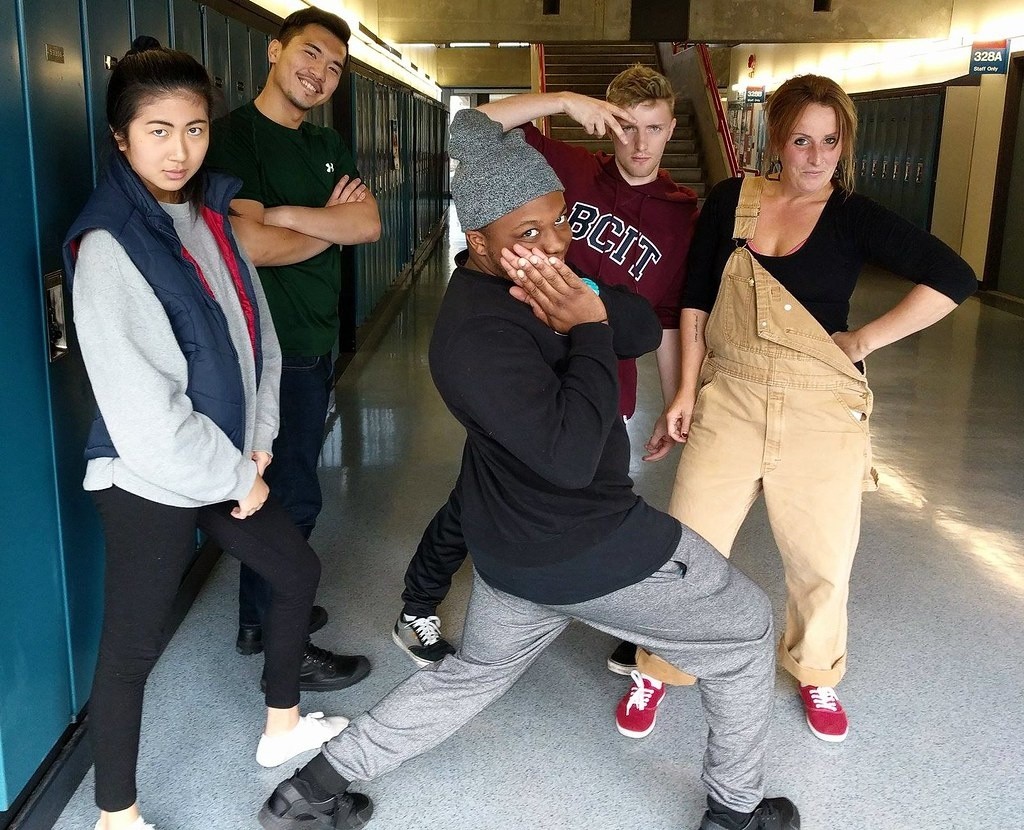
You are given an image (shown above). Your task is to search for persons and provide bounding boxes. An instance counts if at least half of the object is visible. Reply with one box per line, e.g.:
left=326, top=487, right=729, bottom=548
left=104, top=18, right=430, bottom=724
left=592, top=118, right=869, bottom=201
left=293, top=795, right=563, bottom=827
left=64, top=31, right=348, bottom=830
left=200, top=7, right=384, bottom=693
left=392, top=63, right=701, bottom=676
left=617, top=74, right=976, bottom=741
left=257, top=110, right=802, bottom=830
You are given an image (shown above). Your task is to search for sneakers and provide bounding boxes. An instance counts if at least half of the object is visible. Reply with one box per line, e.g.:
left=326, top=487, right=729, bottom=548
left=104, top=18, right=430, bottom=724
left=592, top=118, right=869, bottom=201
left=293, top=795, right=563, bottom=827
left=699, top=795, right=800, bottom=830
left=615, top=671, right=665, bottom=737
left=257, top=768, right=373, bottom=830
left=392, top=610, right=456, bottom=667
left=94, top=815, right=156, bottom=830
left=256, top=712, right=349, bottom=768
left=798, top=680, right=848, bottom=743
left=608, top=637, right=641, bottom=675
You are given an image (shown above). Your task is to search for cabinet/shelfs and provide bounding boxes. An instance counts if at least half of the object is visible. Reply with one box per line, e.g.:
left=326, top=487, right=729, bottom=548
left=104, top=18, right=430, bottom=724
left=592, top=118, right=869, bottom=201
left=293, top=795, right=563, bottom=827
left=0, top=0, right=451, bottom=830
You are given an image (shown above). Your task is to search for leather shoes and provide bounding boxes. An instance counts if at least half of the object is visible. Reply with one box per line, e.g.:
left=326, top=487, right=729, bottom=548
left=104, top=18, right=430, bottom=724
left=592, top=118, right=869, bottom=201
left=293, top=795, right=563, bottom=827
left=260, top=633, right=371, bottom=691
left=236, top=605, right=327, bottom=657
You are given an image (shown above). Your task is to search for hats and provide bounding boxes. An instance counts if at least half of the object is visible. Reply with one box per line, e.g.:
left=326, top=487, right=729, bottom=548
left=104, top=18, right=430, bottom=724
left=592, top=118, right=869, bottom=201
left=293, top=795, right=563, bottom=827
left=447, top=107, right=566, bottom=233
